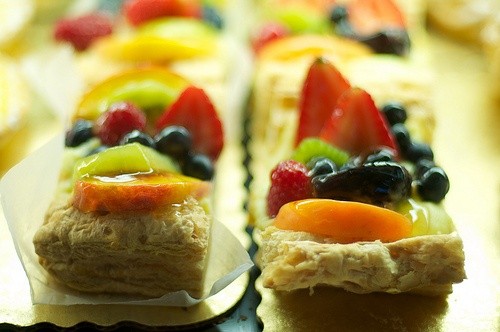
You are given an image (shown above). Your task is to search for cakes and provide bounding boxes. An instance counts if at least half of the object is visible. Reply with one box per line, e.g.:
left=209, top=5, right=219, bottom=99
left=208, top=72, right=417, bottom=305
left=32, top=0, right=467, bottom=298
